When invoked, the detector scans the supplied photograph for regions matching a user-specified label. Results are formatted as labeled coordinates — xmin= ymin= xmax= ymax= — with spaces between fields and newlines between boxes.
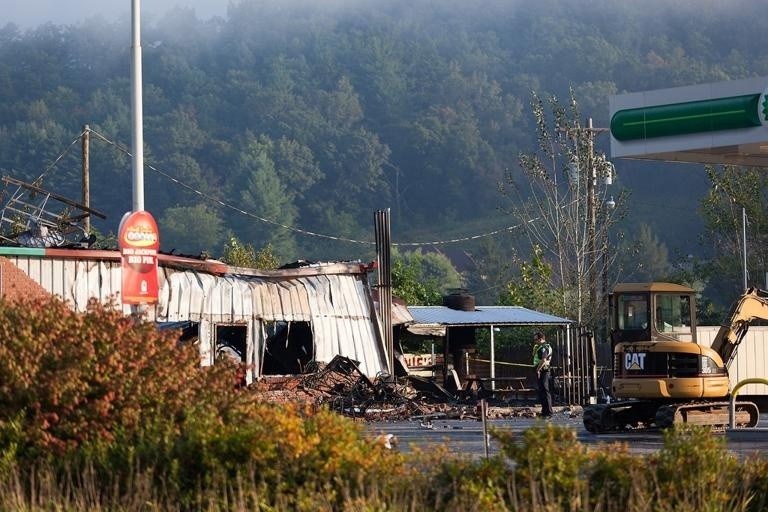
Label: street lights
xmin=600 ymin=194 xmax=616 ymax=302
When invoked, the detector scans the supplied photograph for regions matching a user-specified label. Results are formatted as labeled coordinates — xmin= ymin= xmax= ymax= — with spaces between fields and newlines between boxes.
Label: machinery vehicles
xmin=582 ymin=277 xmax=767 ymax=434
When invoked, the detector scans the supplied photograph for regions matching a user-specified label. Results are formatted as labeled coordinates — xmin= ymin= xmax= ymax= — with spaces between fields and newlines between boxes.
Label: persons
xmin=532 ymin=332 xmax=553 ymax=417
xmin=644 ymin=307 xmax=673 ymax=327
